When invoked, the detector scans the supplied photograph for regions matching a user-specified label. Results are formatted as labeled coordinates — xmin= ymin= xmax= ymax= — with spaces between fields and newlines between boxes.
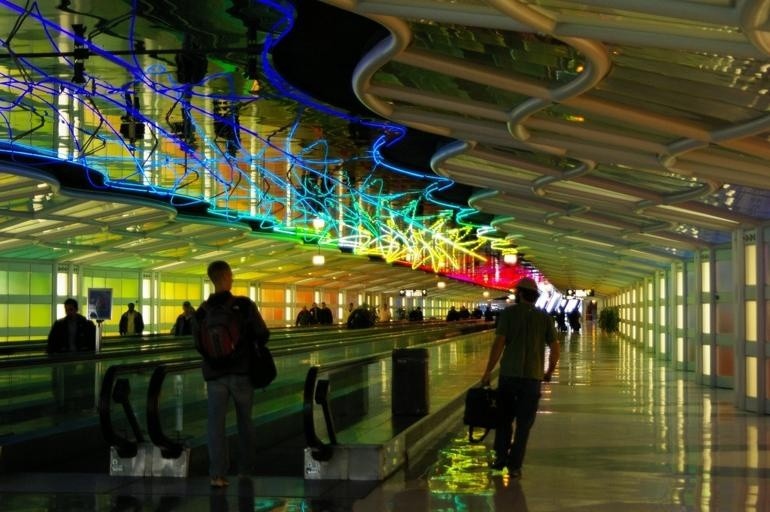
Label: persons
xmin=481 ymin=278 xmax=561 ymax=478
xmin=170 ymin=302 xmax=198 ymax=337
xmin=293 ymin=300 xmax=579 ymax=333
xmin=194 ymin=260 xmax=278 ymax=488
xmin=48 ymin=298 xmax=96 ymax=417
xmin=117 ymin=302 xmax=146 ymax=336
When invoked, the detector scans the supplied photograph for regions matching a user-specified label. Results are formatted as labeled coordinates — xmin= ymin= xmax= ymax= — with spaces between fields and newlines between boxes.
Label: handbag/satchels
xmin=246 ymin=347 xmax=276 ymax=389
xmin=463 ymin=384 xmax=501 ymax=429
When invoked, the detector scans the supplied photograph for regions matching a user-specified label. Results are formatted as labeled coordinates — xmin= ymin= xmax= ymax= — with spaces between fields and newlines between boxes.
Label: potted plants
xmin=599 ymin=306 xmax=620 ymax=339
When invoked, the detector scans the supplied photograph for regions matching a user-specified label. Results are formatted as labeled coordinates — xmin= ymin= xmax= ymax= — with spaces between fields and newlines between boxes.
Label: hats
xmin=518 ymin=277 xmax=538 ymax=291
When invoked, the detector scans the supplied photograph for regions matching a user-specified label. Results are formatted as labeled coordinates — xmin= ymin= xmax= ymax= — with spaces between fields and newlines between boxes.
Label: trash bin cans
xmin=393 ymin=349 xmax=428 ymax=417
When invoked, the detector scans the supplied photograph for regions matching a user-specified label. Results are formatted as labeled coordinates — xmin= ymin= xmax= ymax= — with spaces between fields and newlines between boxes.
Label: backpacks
xmin=201 ymin=298 xmax=245 ymax=363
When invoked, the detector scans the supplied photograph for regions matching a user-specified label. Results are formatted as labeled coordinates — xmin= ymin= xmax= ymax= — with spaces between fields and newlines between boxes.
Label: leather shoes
xmin=508 ymin=468 xmax=521 ymax=477
xmin=491 ymin=460 xmax=508 ymax=471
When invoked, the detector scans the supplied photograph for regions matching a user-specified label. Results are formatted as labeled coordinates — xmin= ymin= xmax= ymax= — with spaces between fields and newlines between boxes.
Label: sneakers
xmin=210 ymin=474 xmax=230 ymax=488
xmin=239 ymin=468 xmax=261 ymax=478
